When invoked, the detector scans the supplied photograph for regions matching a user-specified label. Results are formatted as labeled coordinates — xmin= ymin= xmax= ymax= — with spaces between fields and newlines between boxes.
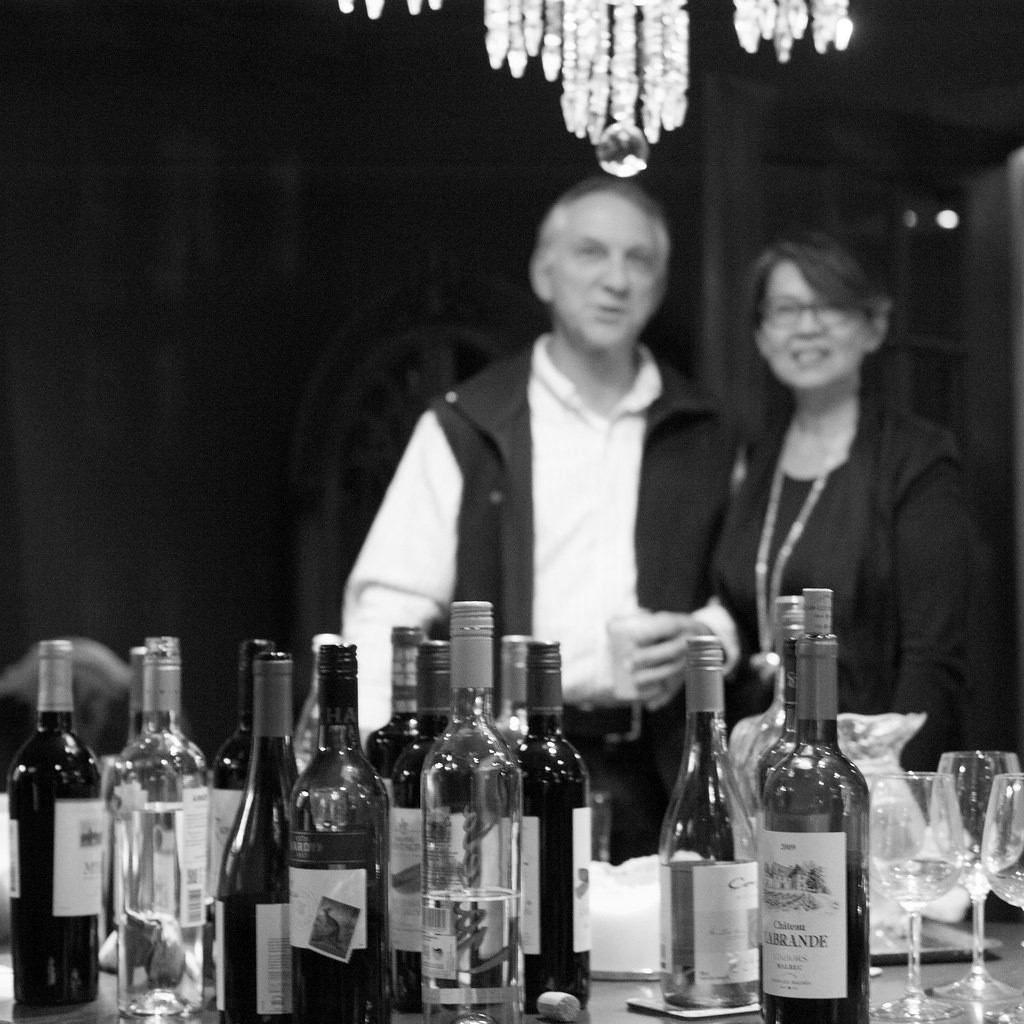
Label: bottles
xmin=658 ymin=589 xmax=870 ymax=1024
xmin=9 ymin=601 xmax=591 ymax=1024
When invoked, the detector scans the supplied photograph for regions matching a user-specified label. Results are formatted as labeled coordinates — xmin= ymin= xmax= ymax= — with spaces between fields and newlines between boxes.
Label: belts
xmin=559 ymin=696 xmax=686 ymax=747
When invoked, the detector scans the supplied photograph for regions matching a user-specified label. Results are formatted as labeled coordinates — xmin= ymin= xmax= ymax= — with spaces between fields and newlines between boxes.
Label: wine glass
xmin=924 ymin=752 xmax=1023 ymax=1007
xmin=981 ymin=773 xmax=1024 ymax=1024
xmin=867 ymin=772 xmax=964 ymax=1022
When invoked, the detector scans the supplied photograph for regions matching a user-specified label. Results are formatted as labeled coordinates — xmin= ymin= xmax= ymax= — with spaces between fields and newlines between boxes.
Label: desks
xmin=0 ymin=922 xmax=1024 ymax=1024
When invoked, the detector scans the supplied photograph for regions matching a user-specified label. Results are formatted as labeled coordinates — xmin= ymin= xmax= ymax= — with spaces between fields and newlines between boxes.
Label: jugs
xmin=838 ymin=711 xmax=926 ymax=943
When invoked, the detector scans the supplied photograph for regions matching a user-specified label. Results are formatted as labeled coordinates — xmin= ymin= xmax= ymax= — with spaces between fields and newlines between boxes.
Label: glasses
xmin=758 ymin=293 xmax=857 ymax=324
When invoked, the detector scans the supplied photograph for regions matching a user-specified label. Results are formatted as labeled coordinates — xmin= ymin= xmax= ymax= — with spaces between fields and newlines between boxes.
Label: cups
xmin=609 ymin=623 xmax=657 ymax=702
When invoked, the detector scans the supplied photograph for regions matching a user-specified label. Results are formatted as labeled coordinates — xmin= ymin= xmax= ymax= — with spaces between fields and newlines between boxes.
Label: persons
xmin=715 ymin=230 xmax=997 ymax=771
xmin=345 ymin=173 xmax=744 ymax=871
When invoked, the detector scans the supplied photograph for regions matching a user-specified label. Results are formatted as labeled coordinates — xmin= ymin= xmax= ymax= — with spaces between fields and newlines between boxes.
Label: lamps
xmin=338 ymin=0 xmax=854 ymax=178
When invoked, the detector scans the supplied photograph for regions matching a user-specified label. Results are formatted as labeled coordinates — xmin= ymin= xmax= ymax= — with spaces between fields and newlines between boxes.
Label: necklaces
xmin=754 ymin=434 xmax=847 ymax=683
